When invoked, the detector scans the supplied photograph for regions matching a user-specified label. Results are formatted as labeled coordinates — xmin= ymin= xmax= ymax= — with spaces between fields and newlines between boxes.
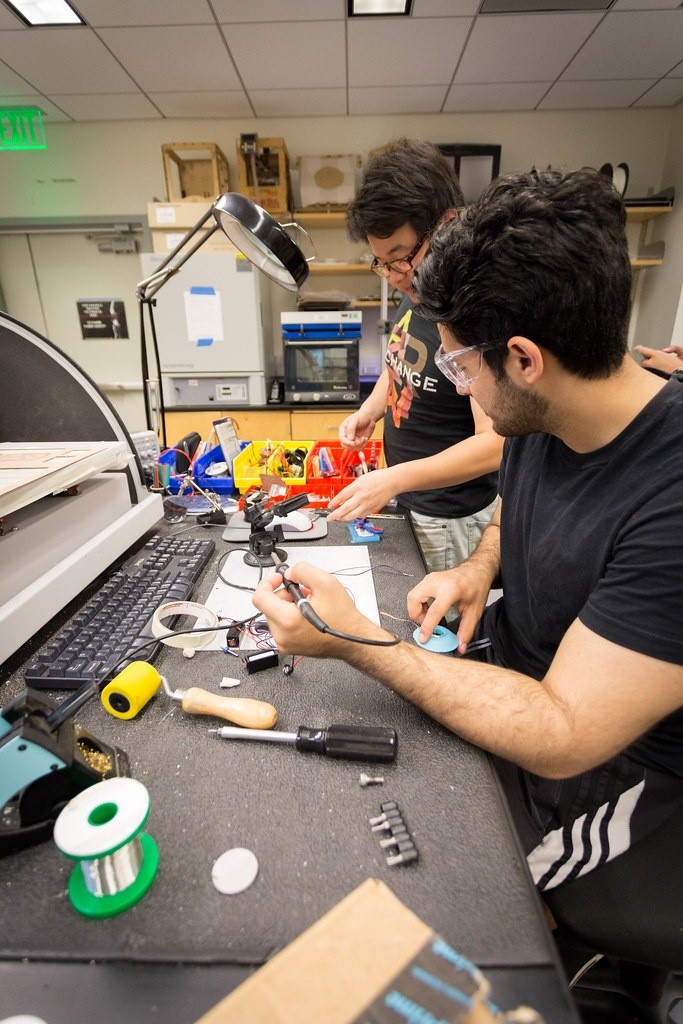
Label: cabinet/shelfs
xmin=270 ymin=213 xmax=400 ymax=307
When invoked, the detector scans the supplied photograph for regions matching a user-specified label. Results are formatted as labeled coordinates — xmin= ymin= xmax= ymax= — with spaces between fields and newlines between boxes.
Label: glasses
xmin=434 ymin=341 xmax=504 ymax=389
xmin=370 ymin=217 xmax=437 ymax=277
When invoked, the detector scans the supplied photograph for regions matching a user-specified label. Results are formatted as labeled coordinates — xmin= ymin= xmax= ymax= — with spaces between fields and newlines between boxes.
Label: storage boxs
xmin=297 ymin=153 xmax=356 ymax=206
xmin=161 ymin=142 xmax=230 ymax=204
xmin=157 ymin=437 xmax=384 ymax=516
xmin=235 ymin=136 xmax=293 ymax=213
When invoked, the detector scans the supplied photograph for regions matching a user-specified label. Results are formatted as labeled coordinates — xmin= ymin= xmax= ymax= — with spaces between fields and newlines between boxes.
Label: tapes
xmin=150 ymin=600 xmax=221 ymax=649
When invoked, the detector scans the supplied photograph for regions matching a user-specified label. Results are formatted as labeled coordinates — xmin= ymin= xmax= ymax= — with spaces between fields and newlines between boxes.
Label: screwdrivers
xmin=206 ymin=724 xmax=398 ymax=763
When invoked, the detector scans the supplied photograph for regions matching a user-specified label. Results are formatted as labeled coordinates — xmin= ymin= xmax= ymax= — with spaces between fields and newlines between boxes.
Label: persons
xmin=326 ymin=139 xmax=500 ymax=623
xmin=252 ymin=171 xmax=683 ymax=1024
xmin=632 ymin=343 xmax=683 ymax=382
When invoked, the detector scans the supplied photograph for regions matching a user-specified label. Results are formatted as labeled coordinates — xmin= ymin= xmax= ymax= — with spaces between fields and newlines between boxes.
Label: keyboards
xmin=25 ymin=535 xmax=217 ymax=689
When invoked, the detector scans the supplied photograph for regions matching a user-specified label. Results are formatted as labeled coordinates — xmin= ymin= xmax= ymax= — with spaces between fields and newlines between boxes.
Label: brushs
xmin=99 ymin=660 xmax=280 ymax=730
